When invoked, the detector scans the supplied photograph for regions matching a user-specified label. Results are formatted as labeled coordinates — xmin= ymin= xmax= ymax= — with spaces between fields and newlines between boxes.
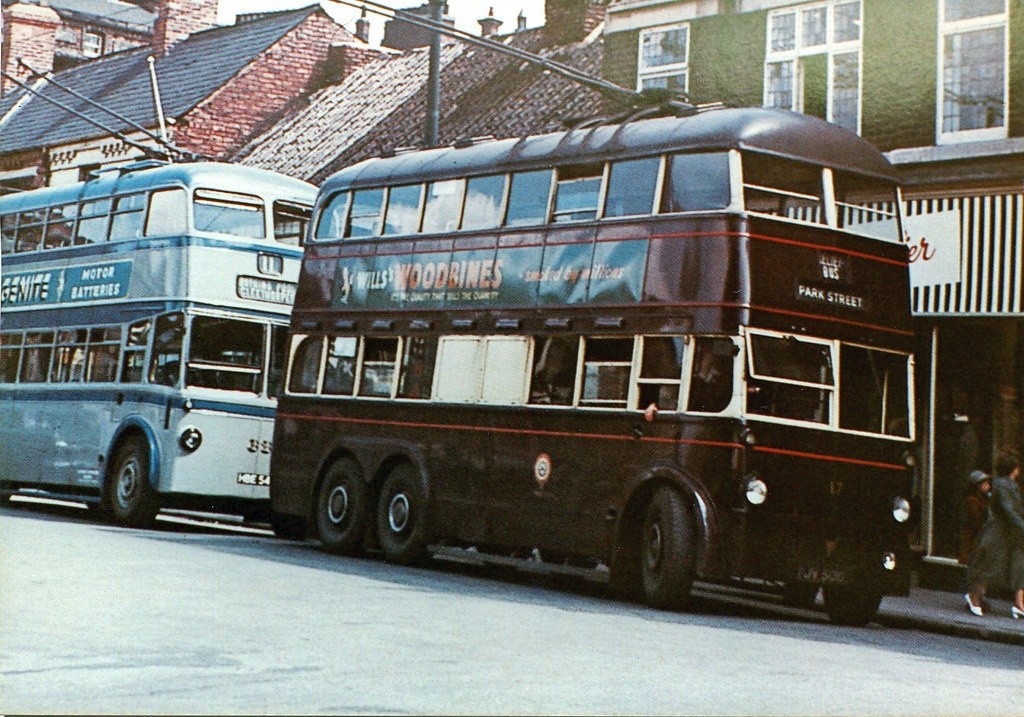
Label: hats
xmin=969 ymin=470 xmax=990 ymax=488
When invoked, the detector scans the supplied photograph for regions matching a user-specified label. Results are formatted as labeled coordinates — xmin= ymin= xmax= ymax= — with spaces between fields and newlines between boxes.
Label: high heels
xmin=965 ymin=593 xmax=983 ymax=616
xmin=1010 ymin=605 xmax=1024 ymax=620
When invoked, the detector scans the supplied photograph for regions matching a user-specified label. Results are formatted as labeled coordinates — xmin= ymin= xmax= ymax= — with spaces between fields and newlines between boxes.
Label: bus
xmin=262 ymin=88 xmax=925 ymax=632
xmin=1 ymin=160 xmax=324 ymax=529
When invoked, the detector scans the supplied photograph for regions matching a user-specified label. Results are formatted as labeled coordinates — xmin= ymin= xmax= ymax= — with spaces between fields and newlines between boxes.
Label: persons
xmin=966 ymin=447 xmax=1024 ymax=621
xmin=960 ymin=470 xmax=991 ymax=609
xmin=533 ymin=332 xmax=723 ymax=421
